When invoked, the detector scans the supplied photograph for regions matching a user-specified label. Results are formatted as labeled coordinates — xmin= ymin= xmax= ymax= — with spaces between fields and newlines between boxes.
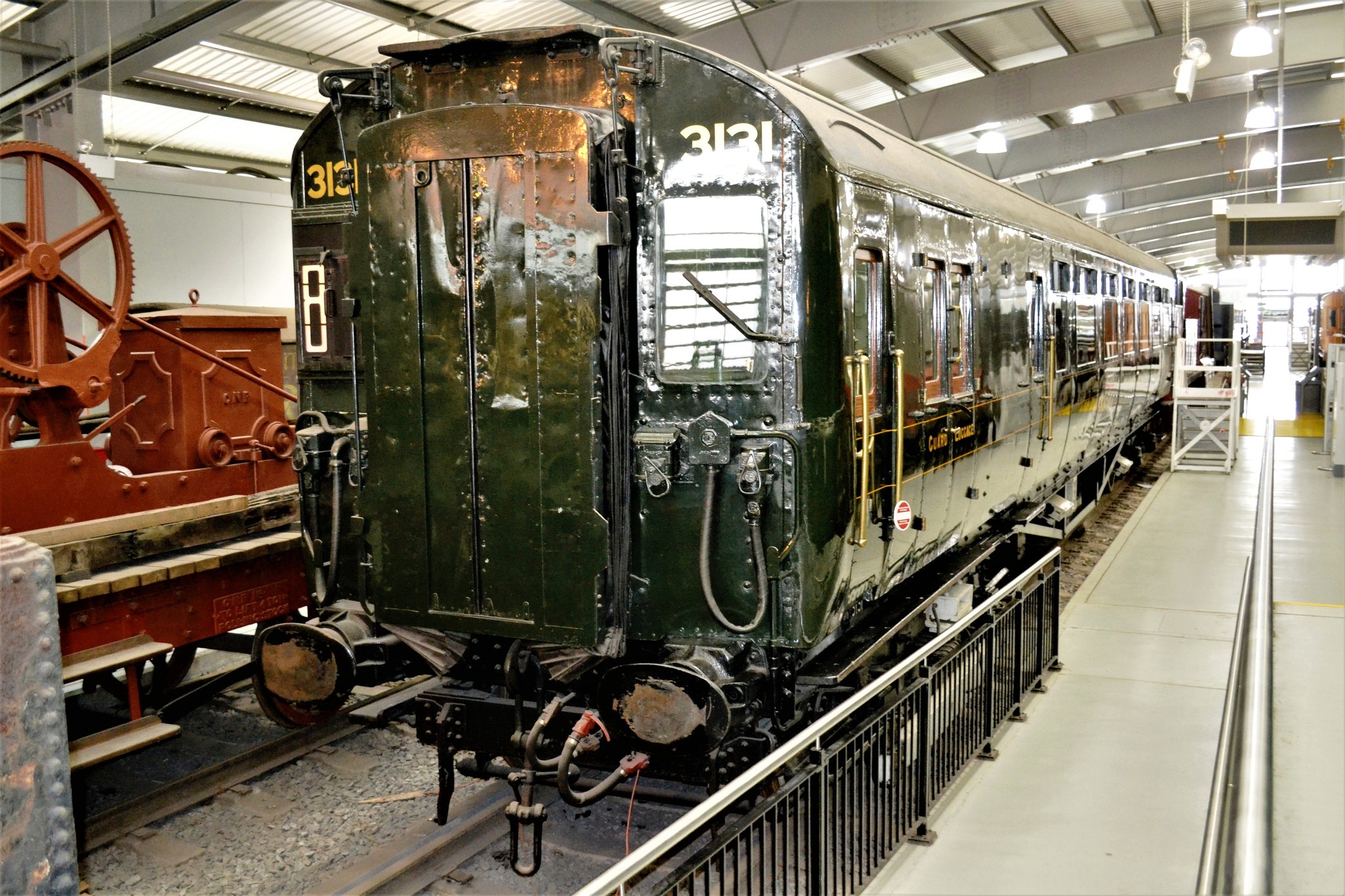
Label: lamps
xmin=1244 ymin=87 xmax=1276 ymax=129
xmin=1173 ymin=38 xmax=1211 ymax=102
xmin=1086 ymin=194 xmax=1106 ymax=214
xmin=1249 ymin=133 xmax=1274 ymax=170
xmin=1230 ymin=19 xmax=1273 ymax=56
xmin=976 ymin=131 xmax=1007 ymax=153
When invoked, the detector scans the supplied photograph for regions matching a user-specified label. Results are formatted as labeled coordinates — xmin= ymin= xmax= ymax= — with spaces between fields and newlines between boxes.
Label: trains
xmin=288 ymin=28 xmax=1246 ymax=878
